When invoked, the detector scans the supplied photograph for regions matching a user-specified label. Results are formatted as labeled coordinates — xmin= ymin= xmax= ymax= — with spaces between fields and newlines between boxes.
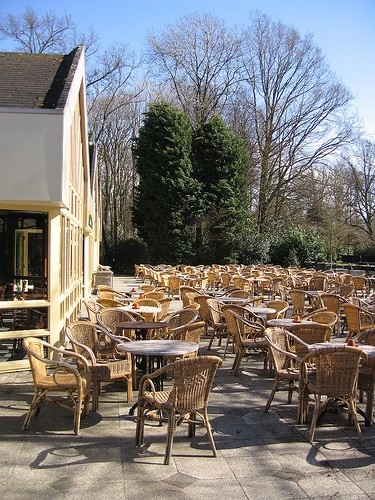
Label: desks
xmin=308 ymin=342 xmax=375 ymax=361
xmin=247 ymin=308 xmax=277 ymax=322
xmin=118 ymin=289 xmax=145 ymax=299
xmin=219 ymin=298 xmax=247 ymax=303
xmin=200 ymin=290 xmax=226 ymax=296
xmin=116 ymin=340 xmax=198 ymax=426
xmin=116 ymin=306 xmax=162 ymax=322
xmin=114 ymin=320 xmax=166 ymax=372
xmin=268 ymin=319 xmax=320 ymax=326
xmin=118 ymin=298 xmax=139 ymax=305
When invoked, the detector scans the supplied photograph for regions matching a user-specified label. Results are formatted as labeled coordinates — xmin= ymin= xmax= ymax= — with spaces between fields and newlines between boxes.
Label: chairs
xmin=0 ymin=263 xmax=375 ymax=465
